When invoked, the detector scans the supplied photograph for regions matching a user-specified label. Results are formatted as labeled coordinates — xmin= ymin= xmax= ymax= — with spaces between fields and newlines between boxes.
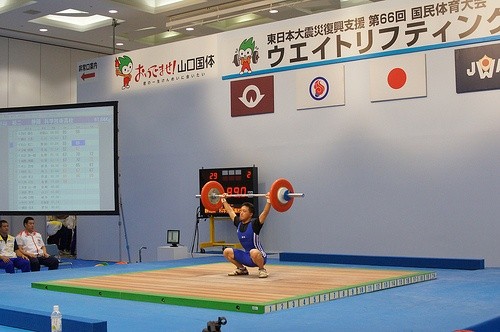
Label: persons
xmin=0 ymin=220 xmax=30 ymax=273
xmin=221 ymin=192 xmax=271 ymax=278
xmin=46 ymin=216 xmax=77 ymax=256
xmin=15 ymin=217 xmax=59 ymax=273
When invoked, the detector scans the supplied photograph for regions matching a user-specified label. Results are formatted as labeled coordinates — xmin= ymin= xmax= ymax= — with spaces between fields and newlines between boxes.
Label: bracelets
xmin=266 ymin=199 xmax=271 ymax=203
xmin=221 ymin=198 xmax=226 ymax=203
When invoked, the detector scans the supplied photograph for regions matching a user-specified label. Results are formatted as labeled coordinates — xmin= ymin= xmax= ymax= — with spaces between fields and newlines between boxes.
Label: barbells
xmin=194 ymin=178 xmax=306 ymax=214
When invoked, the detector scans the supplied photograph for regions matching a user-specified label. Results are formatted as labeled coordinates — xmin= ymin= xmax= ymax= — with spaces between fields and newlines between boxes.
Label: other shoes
xmin=70 ymin=253 xmax=74 ymax=256
xmin=59 ymin=249 xmax=69 ymax=255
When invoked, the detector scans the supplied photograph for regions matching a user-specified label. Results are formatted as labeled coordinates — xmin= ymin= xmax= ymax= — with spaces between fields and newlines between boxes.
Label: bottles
xmin=51 ymin=304 xmax=62 ymax=332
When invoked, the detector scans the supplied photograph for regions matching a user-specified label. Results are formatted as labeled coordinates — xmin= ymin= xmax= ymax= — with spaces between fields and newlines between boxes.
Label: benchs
xmin=14 ymin=244 xmax=72 ymax=271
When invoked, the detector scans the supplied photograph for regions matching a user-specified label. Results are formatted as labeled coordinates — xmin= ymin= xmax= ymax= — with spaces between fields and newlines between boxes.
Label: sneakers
xmin=258 ymin=268 xmax=269 ymax=278
xmin=228 ymin=267 xmax=249 ymax=276
